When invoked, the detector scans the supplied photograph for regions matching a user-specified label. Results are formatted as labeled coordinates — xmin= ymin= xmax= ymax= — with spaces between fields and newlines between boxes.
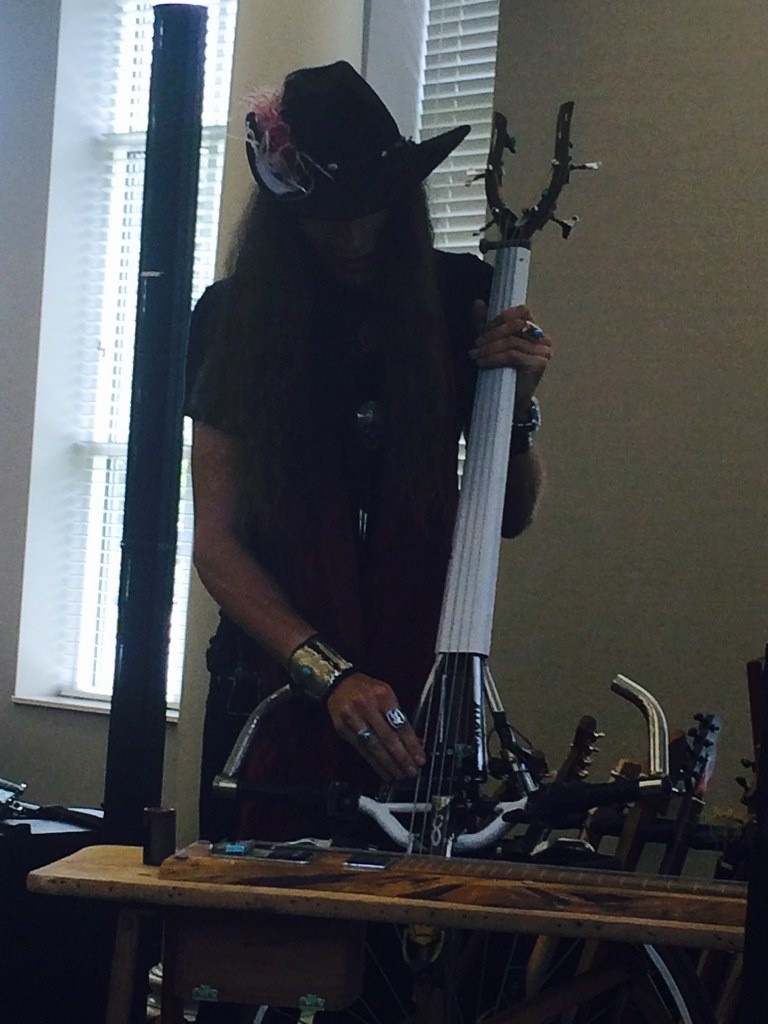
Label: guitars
xmin=355 ymin=97 xmax=601 ymax=985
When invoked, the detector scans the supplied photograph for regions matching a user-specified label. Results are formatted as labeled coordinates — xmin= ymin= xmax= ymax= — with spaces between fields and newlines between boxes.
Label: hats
xmin=243 ymin=61 xmax=470 ymax=220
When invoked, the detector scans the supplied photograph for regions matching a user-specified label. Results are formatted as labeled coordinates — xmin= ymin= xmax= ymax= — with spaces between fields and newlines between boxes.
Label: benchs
xmin=24 ymin=842 xmax=746 ymax=1024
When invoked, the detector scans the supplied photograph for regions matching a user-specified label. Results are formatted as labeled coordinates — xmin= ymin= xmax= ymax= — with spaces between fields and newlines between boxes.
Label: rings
xmin=519 ymin=321 xmax=544 ymax=343
xmin=357 ymin=726 xmax=373 ymax=742
xmin=387 ymin=707 xmax=409 ymax=731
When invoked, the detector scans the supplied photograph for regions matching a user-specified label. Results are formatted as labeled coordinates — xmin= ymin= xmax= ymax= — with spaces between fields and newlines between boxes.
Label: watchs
xmin=512 ymin=393 xmax=543 ymax=433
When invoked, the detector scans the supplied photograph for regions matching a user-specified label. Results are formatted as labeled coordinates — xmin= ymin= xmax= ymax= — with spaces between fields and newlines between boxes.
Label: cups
xmin=142 ymin=806 xmax=177 ymax=865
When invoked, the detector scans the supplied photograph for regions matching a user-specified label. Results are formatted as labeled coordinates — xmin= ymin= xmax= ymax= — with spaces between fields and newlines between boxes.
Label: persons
xmin=177 ymin=62 xmax=549 ymax=840
